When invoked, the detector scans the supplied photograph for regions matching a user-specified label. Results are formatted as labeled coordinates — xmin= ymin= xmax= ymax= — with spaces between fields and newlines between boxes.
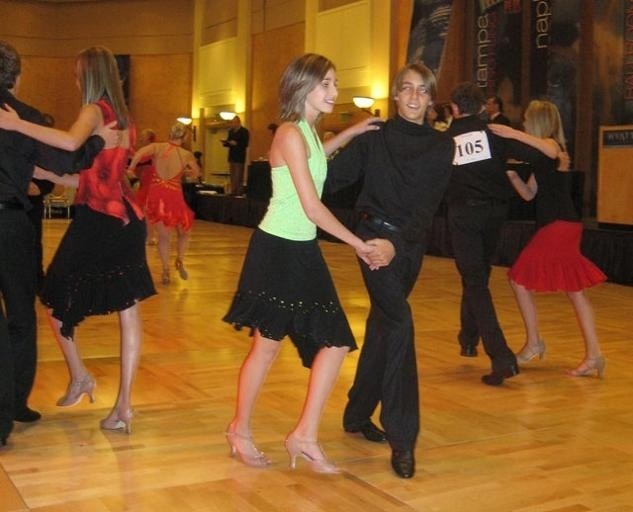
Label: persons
xmin=0 ymin=44 xmax=159 ymax=433
xmin=438 ymin=81 xmax=571 ymax=387
xmin=484 ymin=99 xmax=610 ymax=381
xmin=219 ymin=51 xmax=386 ymax=476
xmin=21 ymin=114 xmax=251 ymax=303
xmin=423 ymin=103 xmax=454 ymax=133
xmin=322 ymin=62 xmax=457 ymax=479
xmin=0 ymin=42 xmax=125 ymax=448
xmin=484 ymin=94 xmax=512 ymax=128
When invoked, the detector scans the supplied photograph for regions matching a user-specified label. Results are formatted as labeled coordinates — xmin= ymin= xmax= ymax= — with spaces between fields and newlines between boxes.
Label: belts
xmin=363 ymin=210 xmax=406 ymax=235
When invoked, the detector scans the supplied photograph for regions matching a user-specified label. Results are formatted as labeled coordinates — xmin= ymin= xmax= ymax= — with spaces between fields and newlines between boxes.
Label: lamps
xmin=352 ymin=96 xmax=379 ymax=117
xmin=177 ymin=118 xmax=195 ymax=142
xmin=219 ymin=112 xmax=239 ymax=121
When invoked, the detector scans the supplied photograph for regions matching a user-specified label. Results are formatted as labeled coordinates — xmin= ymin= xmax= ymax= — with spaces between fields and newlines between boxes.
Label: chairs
xmin=43 ymin=184 xmax=70 ymax=219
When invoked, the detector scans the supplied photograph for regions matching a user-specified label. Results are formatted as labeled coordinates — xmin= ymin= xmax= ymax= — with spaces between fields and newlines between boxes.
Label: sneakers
xmin=160 ymin=264 xmax=170 ymax=284
xmin=173 ymin=259 xmax=188 ymax=281
xmin=15 ymin=406 xmax=41 ymax=425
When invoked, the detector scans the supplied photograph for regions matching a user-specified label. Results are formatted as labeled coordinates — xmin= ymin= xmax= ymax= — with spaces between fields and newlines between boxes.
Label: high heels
xmin=52 ymin=372 xmax=100 ymax=409
xmin=566 ymin=354 xmax=607 ymax=381
xmin=220 ymin=420 xmax=274 ymax=469
xmin=282 ymin=431 xmax=344 ymax=476
xmin=97 ymin=403 xmax=134 ymax=436
xmin=515 ymin=339 xmax=546 ymax=365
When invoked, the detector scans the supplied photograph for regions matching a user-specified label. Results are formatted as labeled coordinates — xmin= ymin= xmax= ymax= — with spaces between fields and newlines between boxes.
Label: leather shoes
xmin=459 ymin=344 xmax=476 ymax=356
xmin=389 ymin=446 xmax=417 ymax=480
xmin=341 ymin=416 xmax=388 ymax=443
xmin=480 ymin=364 xmax=518 ymax=388
